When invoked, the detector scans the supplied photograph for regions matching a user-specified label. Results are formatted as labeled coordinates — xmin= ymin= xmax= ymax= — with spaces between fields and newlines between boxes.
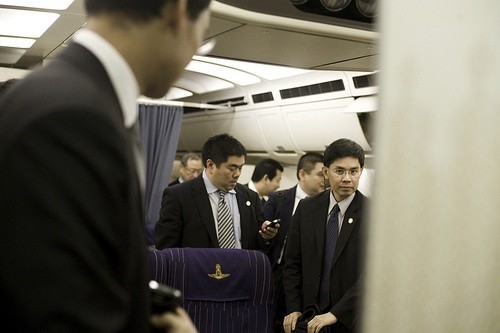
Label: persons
xmin=154 ymin=134 xmax=280 ymax=251
xmin=168 ymin=152 xmax=203 ymax=187
xmin=241 ymin=139 xmax=370 ymax=333
xmin=0 ymin=0 xmax=214 ymax=333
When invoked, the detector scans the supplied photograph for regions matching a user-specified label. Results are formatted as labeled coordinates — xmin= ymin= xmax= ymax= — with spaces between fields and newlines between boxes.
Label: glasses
xmin=185 ymin=167 xmax=203 ymax=174
xmin=332 ymin=169 xmax=362 ymax=177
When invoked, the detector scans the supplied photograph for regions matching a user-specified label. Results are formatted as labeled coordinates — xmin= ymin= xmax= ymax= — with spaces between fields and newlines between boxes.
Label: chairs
xmin=148 ymin=246 xmax=167 ymax=287
xmin=162 ymin=247 xmax=274 ymax=333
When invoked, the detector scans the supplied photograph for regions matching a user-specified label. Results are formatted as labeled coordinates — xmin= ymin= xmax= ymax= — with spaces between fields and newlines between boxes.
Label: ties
xmin=217 ymin=190 xmax=236 ymax=250
xmin=319 ymin=204 xmax=341 ymax=312
xmin=129 ymin=118 xmax=147 ymax=228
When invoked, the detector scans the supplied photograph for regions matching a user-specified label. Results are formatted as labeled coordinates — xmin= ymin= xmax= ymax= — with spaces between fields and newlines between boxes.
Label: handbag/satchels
xmin=274 ymin=304 xmax=330 ymax=333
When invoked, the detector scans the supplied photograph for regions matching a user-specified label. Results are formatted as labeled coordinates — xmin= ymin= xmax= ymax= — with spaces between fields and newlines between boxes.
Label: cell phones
xmin=262 ymin=219 xmax=281 ymax=233
xmin=148 ymin=280 xmax=181 ymax=333
